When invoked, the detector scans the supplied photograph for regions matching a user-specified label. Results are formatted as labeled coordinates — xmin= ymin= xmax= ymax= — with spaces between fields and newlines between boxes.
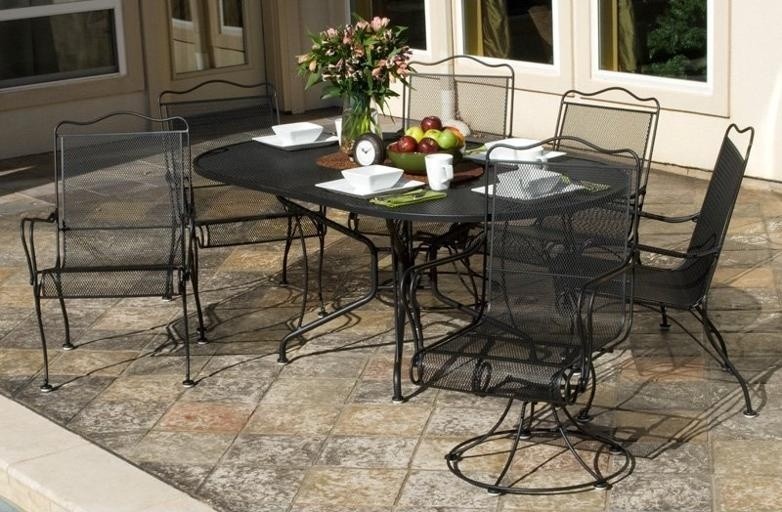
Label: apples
xmin=389 ymin=116 xmax=465 ymax=154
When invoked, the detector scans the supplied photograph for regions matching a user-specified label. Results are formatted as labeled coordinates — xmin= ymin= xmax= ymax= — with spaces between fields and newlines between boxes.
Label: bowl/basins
xmin=339 ymin=164 xmax=403 ymax=191
xmin=499 ymin=167 xmax=562 ymax=196
xmin=386 ymin=141 xmax=466 ymax=176
xmin=483 ymin=136 xmax=542 ymax=160
xmin=271 ymin=121 xmax=323 ymax=144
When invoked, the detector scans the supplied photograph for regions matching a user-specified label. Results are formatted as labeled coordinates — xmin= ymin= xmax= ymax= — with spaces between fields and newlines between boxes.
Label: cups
xmin=334 ymin=117 xmax=345 ymax=146
xmin=424 ymin=153 xmax=454 ymax=191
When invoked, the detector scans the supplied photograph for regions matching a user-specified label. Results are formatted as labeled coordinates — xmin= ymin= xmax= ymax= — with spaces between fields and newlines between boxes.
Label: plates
xmin=251 ymin=133 xmax=340 ymax=153
xmin=314 ymin=176 xmax=424 ymax=201
xmin=464 ymin=146 xmax=567 ymax=166
xmin=471 ymin=184 xmax=586 ymax=203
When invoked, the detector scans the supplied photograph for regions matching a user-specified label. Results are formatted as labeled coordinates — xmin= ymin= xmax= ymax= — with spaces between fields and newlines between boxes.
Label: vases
xmin=338 ymin=89 xmax=387 ymax=155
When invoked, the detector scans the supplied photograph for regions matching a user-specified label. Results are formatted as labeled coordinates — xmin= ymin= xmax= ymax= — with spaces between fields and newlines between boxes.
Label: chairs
xmin=347 ymin=49 xmax=520 ymax=313
xmin=17 ymin=104 xmax=212 ymax=393
xmin=157 ymin=73 xmax=332 ymax=346
xmin=405 ymin=133 xmax=644 ymax=498
xmin=555 ymin=119 xmax=765 ymax=422
xmin=447 ymin=77 xmax=664 ymax=339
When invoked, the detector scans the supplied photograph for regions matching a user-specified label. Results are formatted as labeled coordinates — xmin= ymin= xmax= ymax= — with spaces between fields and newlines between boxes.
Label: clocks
xmin=353 ymin=132 xmax=387 ymax=168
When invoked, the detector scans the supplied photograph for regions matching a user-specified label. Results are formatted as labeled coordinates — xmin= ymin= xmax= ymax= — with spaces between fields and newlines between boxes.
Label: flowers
xmin=292 ymin=12 xmax=415 ymax=145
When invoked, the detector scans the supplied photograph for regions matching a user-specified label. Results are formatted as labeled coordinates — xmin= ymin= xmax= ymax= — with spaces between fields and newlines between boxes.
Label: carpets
xmin=2 ymin=102 xmax=782 ymax=512
